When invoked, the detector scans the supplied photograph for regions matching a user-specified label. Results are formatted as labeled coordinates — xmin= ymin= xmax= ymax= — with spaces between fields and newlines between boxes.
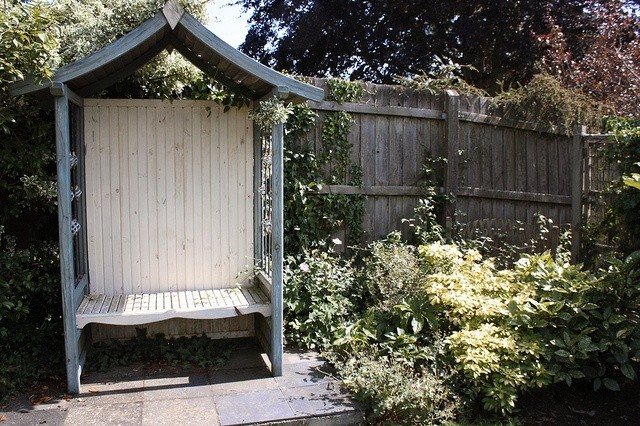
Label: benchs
xmin=73 ymin=268 xmax=277 ymax=328
